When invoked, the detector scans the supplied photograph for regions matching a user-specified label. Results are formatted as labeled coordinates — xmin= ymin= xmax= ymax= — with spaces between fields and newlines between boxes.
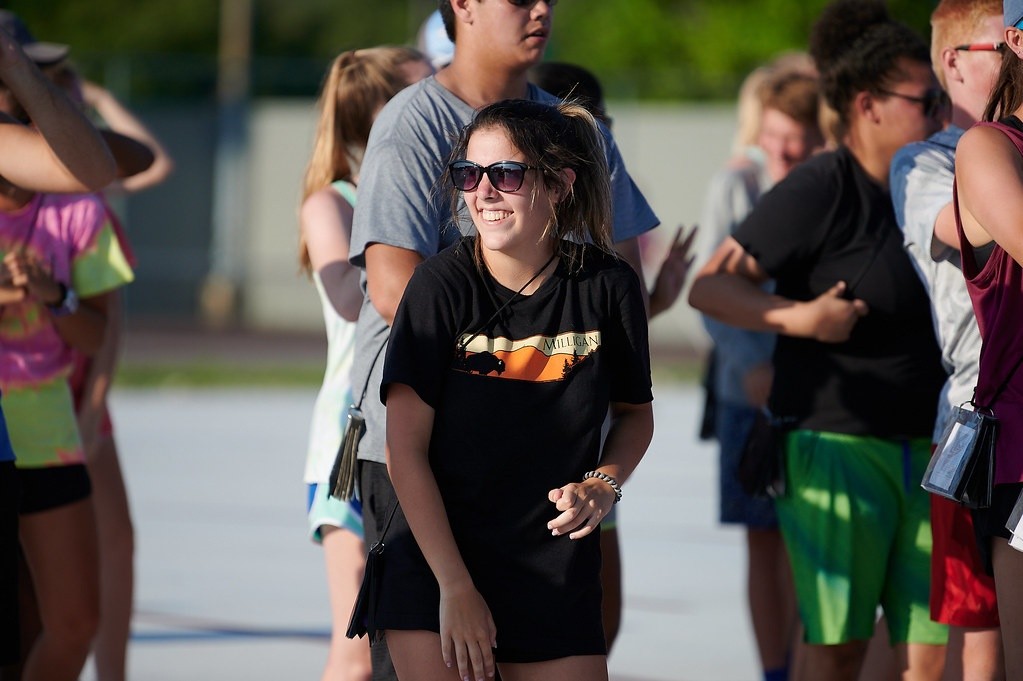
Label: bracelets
xmin=583 ymin=471 xmax=622 ymax=504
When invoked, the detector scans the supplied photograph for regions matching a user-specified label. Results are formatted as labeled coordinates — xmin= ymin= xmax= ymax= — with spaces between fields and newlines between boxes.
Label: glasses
xmin=449 ymin=160 xmax=548 ymax=193
xmin=873 ymin=88 xmax=944 ymax=118
xmin=954 ymin=42 xmax=1009 ymax=62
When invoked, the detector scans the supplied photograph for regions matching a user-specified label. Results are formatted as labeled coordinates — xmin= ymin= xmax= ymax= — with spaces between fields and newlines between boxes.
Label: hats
xmin=1004 ymin=0 xmax=1023 ymax=29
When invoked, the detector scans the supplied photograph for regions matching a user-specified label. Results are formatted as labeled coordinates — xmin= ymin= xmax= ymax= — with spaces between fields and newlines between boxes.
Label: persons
xmin=295 ymin=47 xmax=436 ymax=681
xmin=372 ymin=100 xmax=654 ymax=681
xmin=527 ymin=62 xmax=698 ymax=325
xmin=347 ymin=0 xmax=660 ymax=681
xmin=687 ymin=23 xmax=945 ymax=681
xmin=420 ymin=9 xmax=454 ymax=69
xmin=889 ymin=0 xmax=1004 ymax=681
xmin=952 ymin=0 xmax=1023 ymax=681
xmin=0 ymin=8 xmax=170 ymax=681
xmin=704 ymin=0 xmax=902 ymax=681
